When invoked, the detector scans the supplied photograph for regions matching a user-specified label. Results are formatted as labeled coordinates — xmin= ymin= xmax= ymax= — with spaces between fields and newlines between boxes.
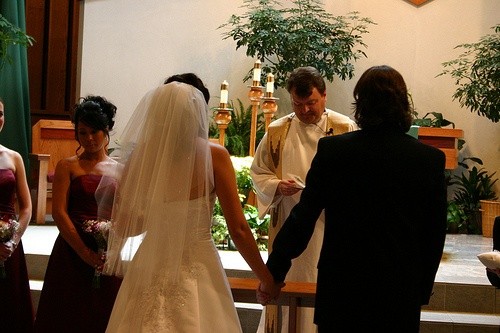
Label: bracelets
xmin=10 ymin=240 xmax=17 ymax=249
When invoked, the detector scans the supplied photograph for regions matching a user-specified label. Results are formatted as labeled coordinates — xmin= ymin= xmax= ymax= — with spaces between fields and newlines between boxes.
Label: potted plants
xmin=411 ymin=112 xmax=465 ymax=169
xmin=436 ymin=22 xmax=500 ymax=245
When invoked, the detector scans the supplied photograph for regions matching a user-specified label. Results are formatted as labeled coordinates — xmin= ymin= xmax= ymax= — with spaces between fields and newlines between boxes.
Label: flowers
xmin=0 ymin=215 xmax=21 ymax=268
xmin=84 ymin=218 xmax=112 ymax=281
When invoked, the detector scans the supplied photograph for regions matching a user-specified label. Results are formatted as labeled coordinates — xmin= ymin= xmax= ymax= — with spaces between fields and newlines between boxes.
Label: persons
xmin=35 ymin=67 xmax=448 ymax=333
xmin=486 ymin=216 xmax=500 ymax=290
xmin=0 ymin=100 xmax=34 ymax=333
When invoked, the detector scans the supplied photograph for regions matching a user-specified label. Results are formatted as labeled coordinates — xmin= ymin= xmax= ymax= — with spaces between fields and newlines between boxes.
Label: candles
xmin=266 ymin=73 xmax=274 ymax=98
xmin=220 ymin=80 xmax=229 ymax=108
xmin=253 ymin=58 xmax=262 ymax=87
